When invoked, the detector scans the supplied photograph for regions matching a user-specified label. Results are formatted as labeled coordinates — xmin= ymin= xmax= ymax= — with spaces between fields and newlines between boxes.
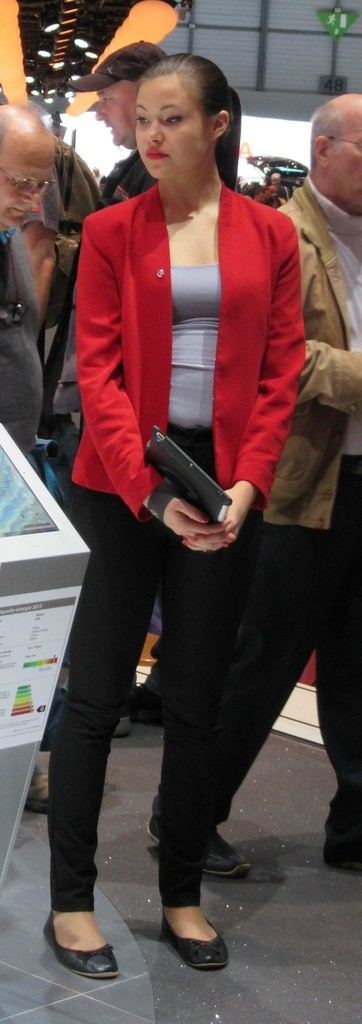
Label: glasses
xmin=0 ymin=167 xmax=57 ymax=196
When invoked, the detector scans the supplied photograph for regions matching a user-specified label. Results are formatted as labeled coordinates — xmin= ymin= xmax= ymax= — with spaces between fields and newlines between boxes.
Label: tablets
xmin=143 ymin=425 xmax=233 ymax=525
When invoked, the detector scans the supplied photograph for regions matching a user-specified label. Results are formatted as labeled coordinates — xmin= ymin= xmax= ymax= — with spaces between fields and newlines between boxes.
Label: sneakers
xmin=147 ymin=824 xmax=254 ymax=877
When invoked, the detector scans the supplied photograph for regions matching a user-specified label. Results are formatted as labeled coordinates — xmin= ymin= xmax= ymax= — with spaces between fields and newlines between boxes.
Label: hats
xmin=67 ymin=41 xmax=168 ymax=92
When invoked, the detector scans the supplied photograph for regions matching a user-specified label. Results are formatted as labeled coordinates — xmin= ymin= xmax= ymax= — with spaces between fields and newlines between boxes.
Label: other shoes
xmin=113 ymin=702 xmax=132 ymax=737
xmin=161 ymin=913 xmax=228 ymax=968
xmin=42 ymin=911 xmax=119 ymax=977
xmin=133 ymin=682 xmax=164 ymax=722
xmin=24 ymin=765 xmax=50 ymax=813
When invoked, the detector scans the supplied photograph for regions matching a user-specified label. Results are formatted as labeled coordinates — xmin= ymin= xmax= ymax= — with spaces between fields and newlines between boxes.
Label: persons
xmin=146 ymin=94 xmax=362 ymax=874
xmin=36 ymin=39 xmax=167 ymax=543
xmin=236 ymin=171 xmax=297 ymax=212
xmin=45 ymin=53 xmax=308 ymax=980
xmin=0 ymin=79 xmax=106 ymax=814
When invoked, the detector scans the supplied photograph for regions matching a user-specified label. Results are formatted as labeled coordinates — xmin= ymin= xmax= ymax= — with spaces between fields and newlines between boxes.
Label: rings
xmin=204 ymin=549 xmax=207 ymax=553
